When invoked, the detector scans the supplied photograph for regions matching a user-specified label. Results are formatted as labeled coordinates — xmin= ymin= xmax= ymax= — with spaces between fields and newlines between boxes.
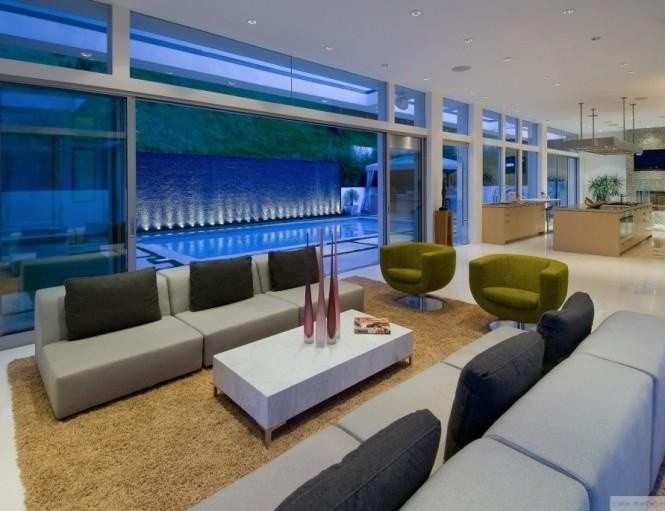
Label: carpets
xmin=8 ymin=274 xmax=498 ymax=511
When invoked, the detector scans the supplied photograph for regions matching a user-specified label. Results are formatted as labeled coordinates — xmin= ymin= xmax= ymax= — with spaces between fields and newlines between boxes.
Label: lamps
xmin=566 ymin=97 xmax=643 ymax=156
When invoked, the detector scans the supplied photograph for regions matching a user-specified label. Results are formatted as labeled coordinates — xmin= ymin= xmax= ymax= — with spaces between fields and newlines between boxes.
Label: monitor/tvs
xmin=633 ymin=149 xmax=665 ymax=171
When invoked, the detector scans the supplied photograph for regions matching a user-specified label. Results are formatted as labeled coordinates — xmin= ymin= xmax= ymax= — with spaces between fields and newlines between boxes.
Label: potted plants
xmin=585 ymin=172 xmax=625 ymax=204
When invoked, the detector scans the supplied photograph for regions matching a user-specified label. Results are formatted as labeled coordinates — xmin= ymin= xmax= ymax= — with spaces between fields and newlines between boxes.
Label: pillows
xmin=268 ymin=246 xmax=319 ymax=292
xmin=64 ymin=266 xmax=162 ymax=341
xmin=272 ymin=290 xmax=594 ymax=511
xmin=189 ymin=255 xmax=254 ymax=312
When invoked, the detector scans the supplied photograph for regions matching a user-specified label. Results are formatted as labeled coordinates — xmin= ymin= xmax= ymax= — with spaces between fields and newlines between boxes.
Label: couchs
xmin=34 ymin=247 xmax=364 ymax=421
xmin=185 ymin=310 xmax=665 ymax=511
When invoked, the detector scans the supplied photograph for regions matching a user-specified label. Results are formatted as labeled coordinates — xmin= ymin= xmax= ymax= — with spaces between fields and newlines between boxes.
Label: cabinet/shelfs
xmin=618 ymin=206 xmax=653 ymax=254
xmin=482 ymin=201 xmax=546 ymax=245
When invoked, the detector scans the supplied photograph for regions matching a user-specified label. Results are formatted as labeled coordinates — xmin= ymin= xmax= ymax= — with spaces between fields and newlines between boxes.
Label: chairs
xmin=379 ymin=241 xmax=456 ymax=311
xmin=469 ymin=253 xmax=568 ymax=332
